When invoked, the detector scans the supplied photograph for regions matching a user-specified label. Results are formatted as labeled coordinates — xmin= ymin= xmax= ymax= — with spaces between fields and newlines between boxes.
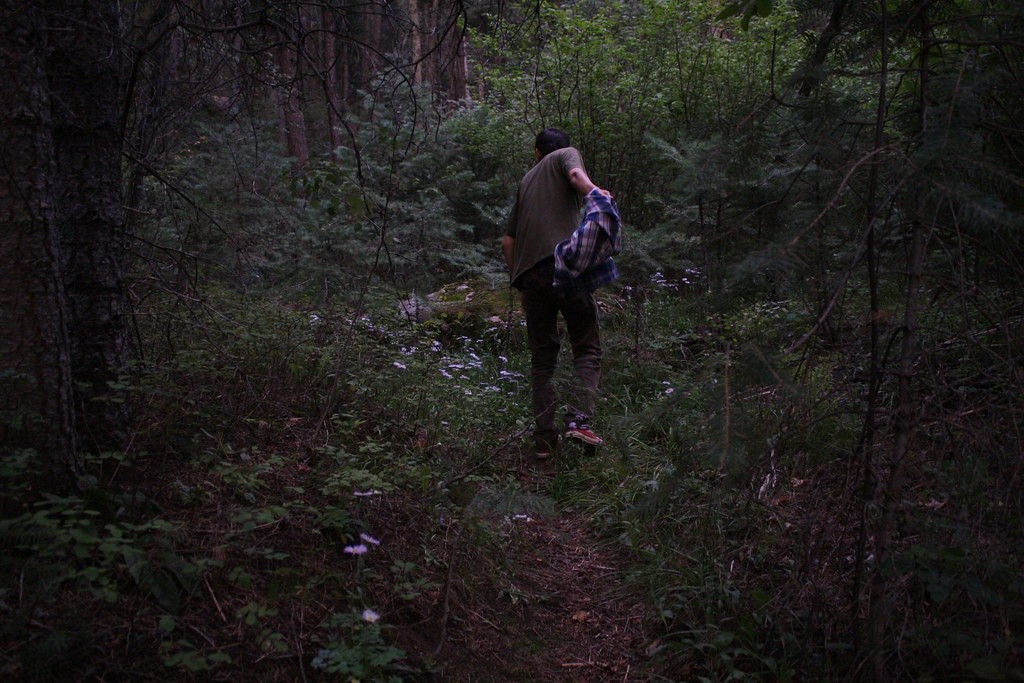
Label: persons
xmin=500 ymin=128 xmax=611 ymax=458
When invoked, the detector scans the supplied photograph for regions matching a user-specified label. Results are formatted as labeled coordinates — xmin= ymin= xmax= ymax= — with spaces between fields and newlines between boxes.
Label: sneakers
xmin=535 ymin=433 xmax=563 ymax=457
xmin=563 ymin=420 xmax=603 ymax=449
